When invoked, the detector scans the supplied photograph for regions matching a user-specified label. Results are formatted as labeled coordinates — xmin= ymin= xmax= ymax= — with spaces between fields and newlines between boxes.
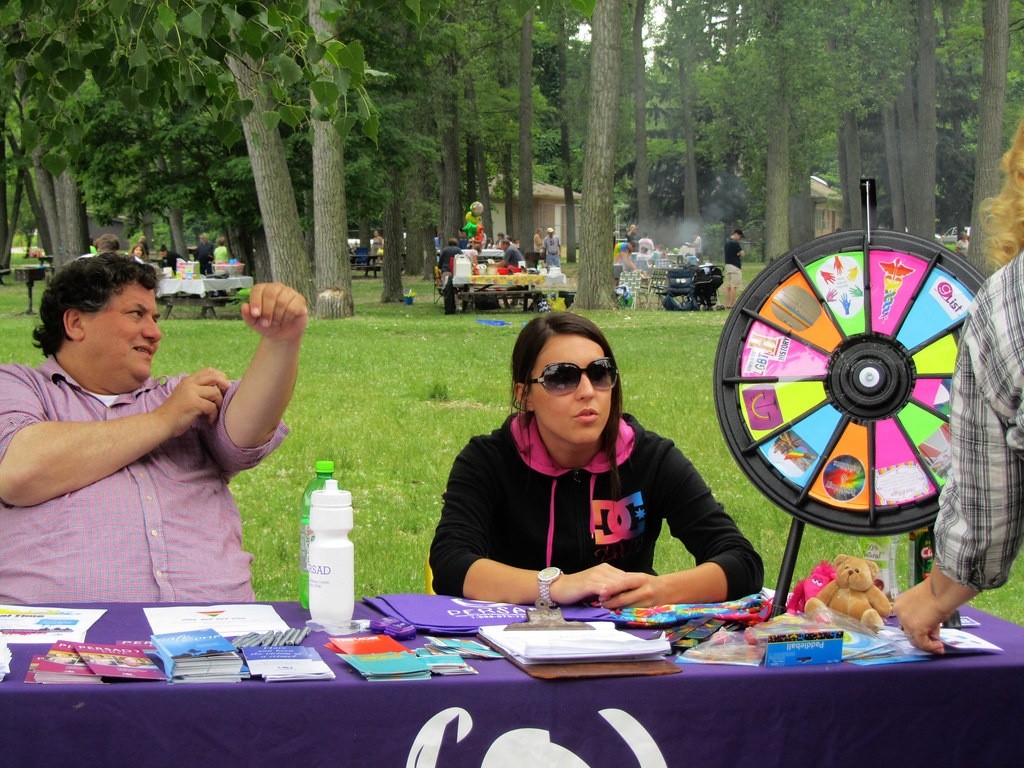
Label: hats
xmin=548 ymin=228 xmax=553 ymax=232
xmin=735 ymin=230 xmax=745 ymax=237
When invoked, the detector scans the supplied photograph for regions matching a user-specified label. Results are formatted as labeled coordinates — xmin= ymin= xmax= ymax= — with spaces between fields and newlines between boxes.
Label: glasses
xmin=528 ymin=357 xmax=619 ymax=396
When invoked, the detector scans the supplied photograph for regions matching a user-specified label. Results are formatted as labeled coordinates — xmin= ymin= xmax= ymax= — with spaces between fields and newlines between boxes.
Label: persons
xmin=612 ymin=223 xmax=702 ymax=264
xmin=955 ymin=231 xmax=969 ymax=255
xmin=436 ymin=227 xmax=562 ymax=315
xmin=88 ymin=234 xmax=230 ymax=298
xmin=0 ymin=247 xmax=308 ymax=604
xmin=426 ymin=312 xmax=763 ymax=610
xmin=351 ymin=230 xmax=383 ymax=278
xmin=892 ymin=120 xmax=1024 ymax=653
xmin=721 ymin=228 xmax=747 ymax=307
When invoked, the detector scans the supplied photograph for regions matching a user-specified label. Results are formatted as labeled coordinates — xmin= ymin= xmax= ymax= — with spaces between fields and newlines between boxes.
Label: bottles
xmin=860 ymin=534 xmax=900 ymax=617
xmin=307 ymin=478 xmax=354 ymax=624
xmin=908 ymin=527 xmax=934 ymax=592
xmin=299 ymin=460 xmax=344 ymax=610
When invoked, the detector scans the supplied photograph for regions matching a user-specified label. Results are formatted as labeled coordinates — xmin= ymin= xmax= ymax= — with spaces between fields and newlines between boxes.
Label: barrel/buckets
xmin=455 ymin=254 xmax=471 ymax=277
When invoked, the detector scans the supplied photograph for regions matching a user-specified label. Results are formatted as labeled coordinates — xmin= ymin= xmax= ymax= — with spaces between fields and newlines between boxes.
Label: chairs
xmin=662 ymin=268 xmax=694 ymax=309
xmin=433 ymin=264 xmax=451 ymax=304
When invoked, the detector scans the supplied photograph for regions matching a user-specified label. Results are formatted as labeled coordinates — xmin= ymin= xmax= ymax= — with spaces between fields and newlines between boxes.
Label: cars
xmin=941 ymin=225 xmax=970 ymax=243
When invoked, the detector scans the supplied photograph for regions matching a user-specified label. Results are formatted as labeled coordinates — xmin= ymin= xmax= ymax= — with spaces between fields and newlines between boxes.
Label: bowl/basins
xmin=498 ymin=268 xmax=509 ymax=275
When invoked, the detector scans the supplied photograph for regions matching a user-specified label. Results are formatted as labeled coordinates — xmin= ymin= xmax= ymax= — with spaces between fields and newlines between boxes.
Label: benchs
xmin=350 ymin=264 xmax=381 ymax=278
xmin=457 ymin=290 xmax=542 ymax=311
xmin=158 ymin=295 xmax=246 ymax=320
xmin=546 ymin=290 xmax=576 ymax=308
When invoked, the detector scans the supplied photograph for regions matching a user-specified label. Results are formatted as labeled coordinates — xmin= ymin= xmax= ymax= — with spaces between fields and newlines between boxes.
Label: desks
xmin=455 ymin=272 xmax=543 ymax=308
xmin=0 ymin=596 xmax=1024 ymax=768
xmin=349 ymin=255 xmax=383 ymax=278
xmin=157 ymin=275 xmax=253 ymax=320
xmin=437 ymin=249 xmax=504 ymax=264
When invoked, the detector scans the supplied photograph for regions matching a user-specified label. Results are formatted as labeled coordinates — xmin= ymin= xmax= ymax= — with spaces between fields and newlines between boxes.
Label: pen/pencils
xmin=240 ymin=634 xmax=265 ymax=648
xmin=294 ymin=626 xmax=312 ymax=646
xmin=261 ymin=631 xmax=281 ymax=647
xmin=286 ymin=629 xmax=301 ymax=646
xmin=277 ymin=628 xmax=296 ymax=647
xmin=235 ymin=633 xmax=259 ymax=647
xmin=249 ymin=630 xmax=274 ymax=647
xmin=232 ymin=632 xmax=255 ymax=646
xmin=272 ymin=632 xmax=283 ymax=647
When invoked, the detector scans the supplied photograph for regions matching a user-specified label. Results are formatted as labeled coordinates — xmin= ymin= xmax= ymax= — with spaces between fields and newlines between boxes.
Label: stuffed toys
xmin=787 ymin=559 xmax=838 ymax=616
xmin=804 ymin=554 xmax=891 ymax=634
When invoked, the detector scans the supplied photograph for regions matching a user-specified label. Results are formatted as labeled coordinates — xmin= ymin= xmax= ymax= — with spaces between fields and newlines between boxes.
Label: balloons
xmin=460 ymin=201 xmax=485 ymax=243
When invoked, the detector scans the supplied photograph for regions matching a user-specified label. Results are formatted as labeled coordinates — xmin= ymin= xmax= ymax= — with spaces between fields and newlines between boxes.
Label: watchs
xmin=537 ymin=567 xmax=564 ymax=606
